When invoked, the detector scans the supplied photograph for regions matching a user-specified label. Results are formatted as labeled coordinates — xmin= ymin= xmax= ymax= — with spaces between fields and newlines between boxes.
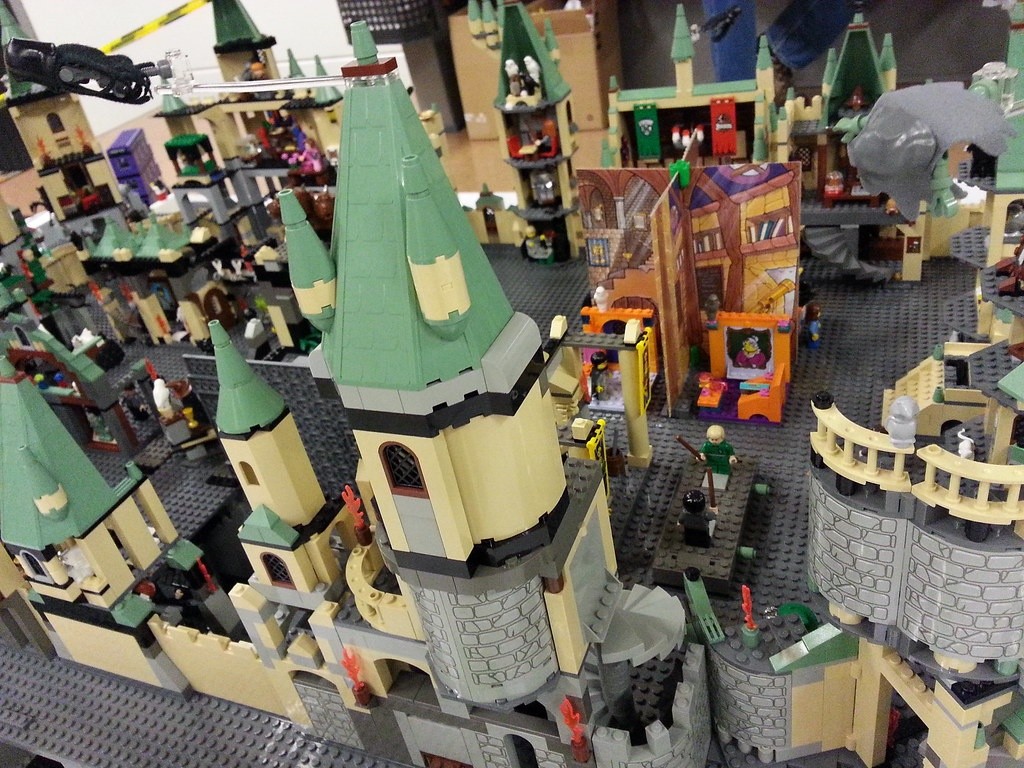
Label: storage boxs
xmin=448 ymin=0 xmax=624 ymax=141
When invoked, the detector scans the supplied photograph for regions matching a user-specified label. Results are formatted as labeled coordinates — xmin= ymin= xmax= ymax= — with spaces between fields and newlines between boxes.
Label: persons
xmin=736 ymin=338 xmax=765 ymax=369
xmin=298 ymin=139 xmax=324 ymax=177
xmin=159 ymin=566 xmax=210 ymax=633
xmin=704 ymin=0 xmax=850 ymax=115
xmin=242 ymin=63 xmax=272 ymax=100
xmin=590 ymin=352 xmax=614 ymax=401
xmin=696 ymin=425 xmax=738 ymax=475
xmin=679 ymin=489 xmax=718 ymax=549
xmin=535 ymin=120 xmax=557 ymax=153
xmin=804 ymin=303 xmax=821 ymax=351
xmin=119 ymin=382 xmax=150 ymax=421
